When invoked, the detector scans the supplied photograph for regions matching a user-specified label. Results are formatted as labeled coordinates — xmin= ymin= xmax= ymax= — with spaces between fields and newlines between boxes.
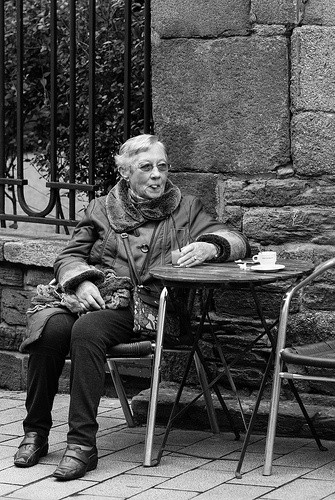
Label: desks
xmin=150 ymin=256 xmax=327 ymax=478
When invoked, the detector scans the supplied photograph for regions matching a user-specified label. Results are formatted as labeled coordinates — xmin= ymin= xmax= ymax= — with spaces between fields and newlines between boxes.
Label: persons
xmin=14 ymin=133 xmax=250 ymax=478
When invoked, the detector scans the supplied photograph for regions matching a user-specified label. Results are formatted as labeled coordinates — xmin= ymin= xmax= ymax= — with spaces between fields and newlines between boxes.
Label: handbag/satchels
xmin=132 ymin=287 xmax=182 ymax=345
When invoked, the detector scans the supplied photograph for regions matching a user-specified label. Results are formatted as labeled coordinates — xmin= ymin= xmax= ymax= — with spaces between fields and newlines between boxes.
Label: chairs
xmin=49 ymin=278 xmax=220 ymax=467
xmin=263 ymin=257 xmax=335 ymax=476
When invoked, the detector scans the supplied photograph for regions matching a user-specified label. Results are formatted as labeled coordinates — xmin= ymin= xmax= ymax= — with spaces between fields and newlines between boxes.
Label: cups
xmin=170 ymin=227 xmax=190 ymax=268
xmin=252 ymin=251 xmax=277 ymax=268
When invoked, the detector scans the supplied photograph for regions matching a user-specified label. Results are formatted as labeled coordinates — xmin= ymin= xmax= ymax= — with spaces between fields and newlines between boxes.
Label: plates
xmin=251 ymin=263 xmax=285 ymax=272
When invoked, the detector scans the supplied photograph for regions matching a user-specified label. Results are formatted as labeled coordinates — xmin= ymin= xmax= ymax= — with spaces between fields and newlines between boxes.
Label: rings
xmin=194 ymin=256 xmax=197 ymax=260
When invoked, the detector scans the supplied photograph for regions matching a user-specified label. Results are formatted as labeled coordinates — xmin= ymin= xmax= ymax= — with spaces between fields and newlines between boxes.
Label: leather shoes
xmin=52 ymin=444 xmax=98 ymax=480
xmin=13 ymin=431 xmax=48 ymax=468
